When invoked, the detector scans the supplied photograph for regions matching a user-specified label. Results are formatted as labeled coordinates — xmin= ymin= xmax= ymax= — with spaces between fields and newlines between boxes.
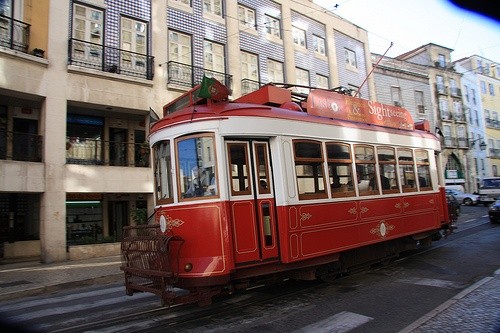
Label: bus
xmin=120 ymin=75 xmax=458 ymax=304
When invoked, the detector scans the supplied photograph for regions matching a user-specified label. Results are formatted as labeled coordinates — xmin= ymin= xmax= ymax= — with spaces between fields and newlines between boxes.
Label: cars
xmin=489 ymin=199 xmax=500 ymax=225
xmin=445 ymin=188 xmax=480 ymax=206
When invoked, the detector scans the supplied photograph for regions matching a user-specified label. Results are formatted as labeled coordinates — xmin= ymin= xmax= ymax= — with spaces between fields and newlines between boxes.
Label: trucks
xmin=478 ymin=177 xmax=500 ymax=205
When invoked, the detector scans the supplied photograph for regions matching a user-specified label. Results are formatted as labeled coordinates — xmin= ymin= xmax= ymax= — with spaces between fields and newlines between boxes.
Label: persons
xmin=331 ymin=164 xmax=427 ymax=192
xmin=180 ymin=164 xmax=216 ymax=197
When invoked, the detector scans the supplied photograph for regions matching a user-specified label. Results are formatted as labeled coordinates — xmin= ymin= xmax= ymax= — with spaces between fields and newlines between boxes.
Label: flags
xmin=197 ymin=75 xmax=227 ymax=102
xmin=148 ymin=108 xmax=160 ymax=124
